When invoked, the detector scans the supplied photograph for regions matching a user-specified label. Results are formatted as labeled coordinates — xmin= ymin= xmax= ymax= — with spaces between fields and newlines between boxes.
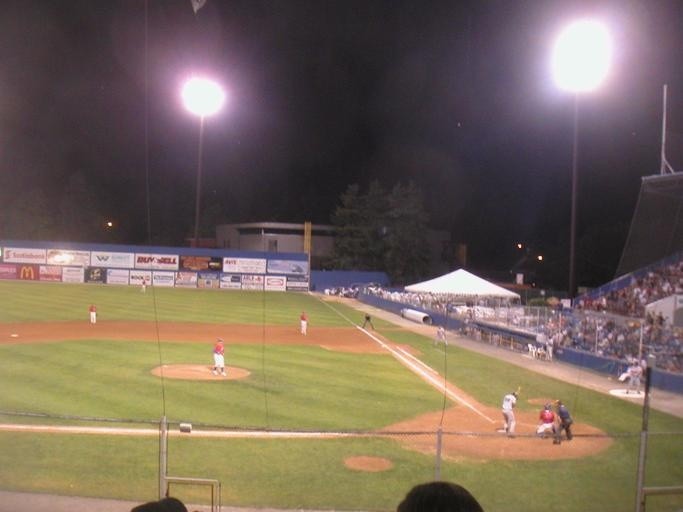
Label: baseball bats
xmin=517 ymin=386 xmax=520 ymax=396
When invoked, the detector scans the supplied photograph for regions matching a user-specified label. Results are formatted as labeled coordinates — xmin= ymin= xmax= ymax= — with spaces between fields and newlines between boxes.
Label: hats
xmin=555 ymin=400 xmax=561 ymax=404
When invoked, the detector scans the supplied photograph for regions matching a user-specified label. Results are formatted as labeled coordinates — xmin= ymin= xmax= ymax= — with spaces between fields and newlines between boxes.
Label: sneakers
xmin=212 ymin=370 xmax=226 ymax=376
xmin=553 ymin=440 xmax=560 ymax=444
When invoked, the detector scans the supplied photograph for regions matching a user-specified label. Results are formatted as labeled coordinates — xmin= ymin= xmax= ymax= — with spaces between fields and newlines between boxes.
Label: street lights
xmin=181 ymin=76 xmax=226 ymax=249
xmin=550 ymin=23 xmax=615 ymax=299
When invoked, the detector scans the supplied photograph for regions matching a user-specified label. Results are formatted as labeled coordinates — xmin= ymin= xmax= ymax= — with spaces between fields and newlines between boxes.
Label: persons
xmin=551 ymin=398 xmax=574 ymax=445
xmin=497 ymin=390 xmax=519 ymax=438
xmin=88 ymin=301 xmax=98 ymax=325
xmin=211 ymin=335 xmax=226 ymax=377
xmin=625 ymin=358 xmax=644 ymax=395
xmin=433 ymin=324 xmax=448 ymax=346
xmin=361 ymin=312 xmax=375 ymax=330
xmin=299 ymin=310 xmax=310 ymax=336
xmin=139 ymin=276 xmax=146 ymax=292
xmin=535 ymin=400 xmax=557 ymax=438
xmin=322 ymin=281 xmax=485 ymax=315
xmin=395 ymin=480 xmax=484 ymax=512
xmin=527 ymin=261 xmax=683 ymax=384
